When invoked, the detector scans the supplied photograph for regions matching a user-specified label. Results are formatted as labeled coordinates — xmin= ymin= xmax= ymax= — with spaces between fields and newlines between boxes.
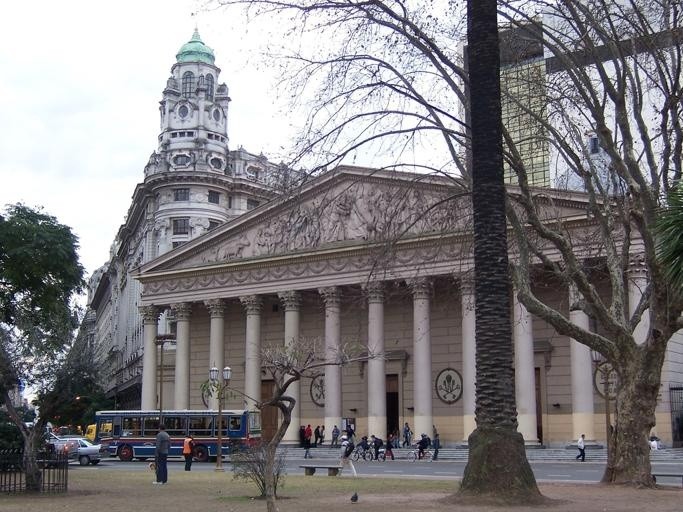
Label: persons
xmin=432 ymin=434 xmax=441 ymax=460
xmin=403 ymin=423 xmax=412 ymax=448
xmin=182 ymin=435 xmax=195 ymax=471
xmin=650 ymin=431 xmax=661 ymax=449
xmin=356 ymin=436 xmax=370 ymax=460
xmin=344 ymin=425 xmax=355 ymax=442
xmin=152 ymin=424 xmax=171 ymax=485
xmin=370 ymin=435 xmax=384 ymax=460
xmin=576 ymin=434 xmax=586 ymax=462
xmin=345 ymin=438 xmax=354 ymax=457
xmin=416 ymin=433 xmax=429 ymax=460
xmin=319 ymin=425 xmax=325 ymax=445
xmin=314 ymin=426 xmax=322 ymax=447
xmin=384 ymin=434 xmax=394 ymax=460
xmin=304 ymin=435 xmax=312 ymax=458
xmin=331 ymin=425 xmax=339 ymax=448
xmin=299 ymin=426 xmax=305 ymax=447
xmin=175 ymin=419 xmax=238 ymax=436
xmin=433 ymin=425 xmax=437 ymax=437
xmin=306 ymin=424 xmax=312 ymax=446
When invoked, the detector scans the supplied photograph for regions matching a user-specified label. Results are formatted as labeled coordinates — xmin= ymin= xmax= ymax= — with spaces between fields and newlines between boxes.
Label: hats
xmin=340 ymin=435 xmax=348 ymax=440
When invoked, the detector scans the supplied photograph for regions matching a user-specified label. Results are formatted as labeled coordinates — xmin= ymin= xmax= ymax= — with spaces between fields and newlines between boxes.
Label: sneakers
xmin=153 ymin=481 xmax=167 ymax=484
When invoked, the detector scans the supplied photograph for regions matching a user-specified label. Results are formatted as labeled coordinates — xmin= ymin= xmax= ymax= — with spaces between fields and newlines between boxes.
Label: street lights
xmin=589 ymin=347 xmax=614 ymax=459
xmin=75 ymin=383 xmax=117 ymax=410
xmin=209 ymin=361 xmax=232 ymax=472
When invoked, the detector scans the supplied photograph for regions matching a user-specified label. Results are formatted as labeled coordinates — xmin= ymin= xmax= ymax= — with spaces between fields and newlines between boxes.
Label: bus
xmin=84 ymin=410 xmax=263 ymax=462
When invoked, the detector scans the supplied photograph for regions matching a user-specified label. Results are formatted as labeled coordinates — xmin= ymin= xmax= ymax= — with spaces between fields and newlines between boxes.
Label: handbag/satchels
xmin=345 ymin=443 xmax=354 ymax=457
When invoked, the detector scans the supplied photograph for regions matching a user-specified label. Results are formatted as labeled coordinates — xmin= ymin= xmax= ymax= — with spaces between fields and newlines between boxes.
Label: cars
xmin=52 ymin=438 xmax=104 ymax=466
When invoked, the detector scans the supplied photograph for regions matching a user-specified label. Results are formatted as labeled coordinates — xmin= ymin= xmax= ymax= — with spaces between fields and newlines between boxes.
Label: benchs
xmin=298 ymin=465 xmax=343 ymax=476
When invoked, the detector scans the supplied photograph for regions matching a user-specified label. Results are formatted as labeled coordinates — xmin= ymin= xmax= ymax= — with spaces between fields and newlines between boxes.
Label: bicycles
xmin=406 ymin=445 xmax=433 ymax=463
xmin=350 ymin=443 xmax=386 ymax=463
